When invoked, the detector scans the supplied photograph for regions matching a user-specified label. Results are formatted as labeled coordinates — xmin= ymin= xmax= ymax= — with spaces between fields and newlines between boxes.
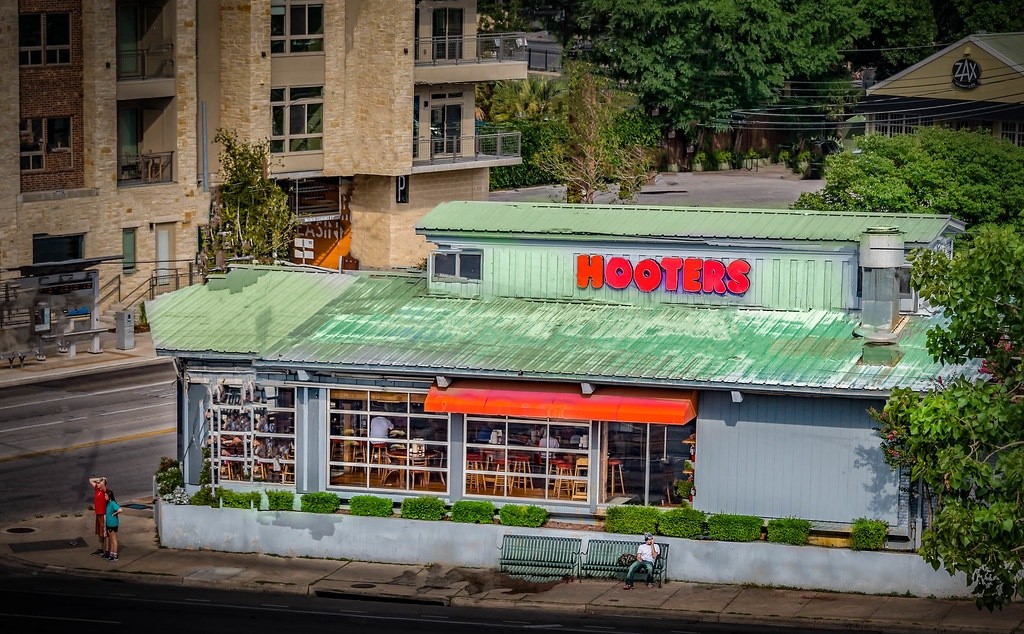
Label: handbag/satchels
xmin=617 ymin=553 xmax=636 ymax=567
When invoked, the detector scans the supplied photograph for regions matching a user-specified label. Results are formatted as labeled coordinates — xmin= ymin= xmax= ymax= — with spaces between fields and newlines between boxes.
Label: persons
xmin=88 ymin=477 xmax=111 ymax=559
xmin=369 ymin=409 xmax=396 ymax=448
xmin=478 ymin=422 xmax=496 ymax=440
xmin=103 ymin=490 xmax=123 ymax=561
xmin=622 ymin=534 xmax=661 ymax=589
xmin=539 ymin=429 xmax=560 ymax=463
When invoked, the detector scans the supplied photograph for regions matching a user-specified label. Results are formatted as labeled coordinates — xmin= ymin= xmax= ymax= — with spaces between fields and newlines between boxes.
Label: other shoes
xmin=101 ymin=551 xmax=110 ymax=559
xmin=623 ymin=584 xmax=634 ymax=589
xmin=91 ymin=548 xmax=104 ymax=556
xmin=113 ymin=553 xmax=119 ymax=561
xmin=648 ymin=583 xmax=653 ymax=588
xmin=107 ymin=552 xmax=114 ymax=560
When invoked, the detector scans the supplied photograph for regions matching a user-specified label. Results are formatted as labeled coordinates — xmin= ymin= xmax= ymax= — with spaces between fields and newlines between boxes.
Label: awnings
xmin=422 ymin=375 xmax=698 ymax=425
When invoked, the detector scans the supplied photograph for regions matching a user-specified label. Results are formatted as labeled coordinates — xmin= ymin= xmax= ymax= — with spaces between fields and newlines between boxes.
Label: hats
xmin=645 ymin=532 xmax=653 ymax=541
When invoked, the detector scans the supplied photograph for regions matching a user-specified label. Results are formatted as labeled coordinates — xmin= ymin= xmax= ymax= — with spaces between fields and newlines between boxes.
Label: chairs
xmin=409 ymin=458 xmax=429 ymax=490
xmin=571 ymin=457 xmax=588 ymax=501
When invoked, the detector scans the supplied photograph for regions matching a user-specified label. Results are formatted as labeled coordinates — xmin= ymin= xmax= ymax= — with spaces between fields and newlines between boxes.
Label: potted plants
xmin=717 ymin=152 xmax=733 ymax=169
xmin=692 ymin=152 xmax=707 ymax=171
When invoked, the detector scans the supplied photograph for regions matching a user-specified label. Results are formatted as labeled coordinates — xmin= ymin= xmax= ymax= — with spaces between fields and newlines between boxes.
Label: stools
xmin=0 ymin=351 xmax=18 ymax=370
xmin=16 ymin=347 xmax=40 ymax=369
xmin=221 ymin=443 xmax=627 ymax=502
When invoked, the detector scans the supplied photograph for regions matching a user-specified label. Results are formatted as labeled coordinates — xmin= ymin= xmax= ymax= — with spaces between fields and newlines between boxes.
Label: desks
xmin=386 ymin=448 xmax=438 ymax=488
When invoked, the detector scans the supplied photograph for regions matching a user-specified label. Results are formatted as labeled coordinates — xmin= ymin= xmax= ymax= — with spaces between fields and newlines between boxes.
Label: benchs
xmin=497 ymin=534 xmax=582 ymax=582
xmin=580 ymin=539 xmax=669 ymax=588
